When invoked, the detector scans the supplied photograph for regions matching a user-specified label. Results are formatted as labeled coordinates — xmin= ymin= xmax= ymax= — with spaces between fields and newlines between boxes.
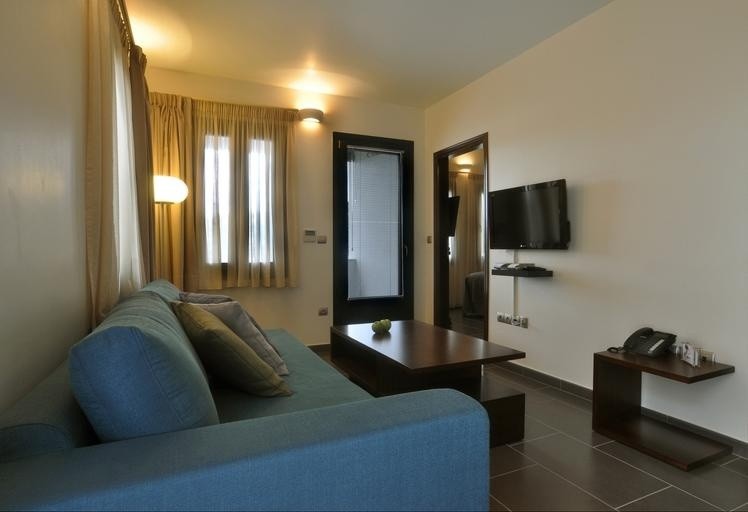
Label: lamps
xmin=296 ymin=107 xmax=325 ymax=124
xmin=152 ymin=174 xmax=190 ymax=281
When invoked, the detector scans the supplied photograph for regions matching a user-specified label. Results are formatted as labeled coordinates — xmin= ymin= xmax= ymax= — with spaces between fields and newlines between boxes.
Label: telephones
xmin=623 ymin=327 xmax=677 ymax=358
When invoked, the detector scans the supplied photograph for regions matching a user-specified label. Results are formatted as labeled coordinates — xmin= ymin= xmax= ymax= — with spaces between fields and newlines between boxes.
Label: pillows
xmin=177 ymin=289 xmax=283 ymax=360
xmin=193 ymin=297 xmax=291 ymax=379
xmin=163 ymin=298 xmax=293 ymax=398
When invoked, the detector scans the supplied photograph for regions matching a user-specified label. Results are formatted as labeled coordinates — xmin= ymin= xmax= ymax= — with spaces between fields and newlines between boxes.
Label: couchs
xmin=1 ymin=278 xmax=492 ymax=512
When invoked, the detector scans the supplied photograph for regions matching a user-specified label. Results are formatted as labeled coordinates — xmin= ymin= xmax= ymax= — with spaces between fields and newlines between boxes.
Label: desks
xmin=587 ymin=345 xmax=737 ymax=475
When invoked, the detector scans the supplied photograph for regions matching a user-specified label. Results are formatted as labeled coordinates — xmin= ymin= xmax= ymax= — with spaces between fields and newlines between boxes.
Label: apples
xmin=372 ymin=319 xmax=391 ymax=333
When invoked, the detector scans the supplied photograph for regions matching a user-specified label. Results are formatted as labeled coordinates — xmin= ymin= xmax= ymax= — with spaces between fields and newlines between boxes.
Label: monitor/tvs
xmin=488 ymin=179 xmax=569 ymax=249
xmin=449 ymin=195 xmax=460 ymax=237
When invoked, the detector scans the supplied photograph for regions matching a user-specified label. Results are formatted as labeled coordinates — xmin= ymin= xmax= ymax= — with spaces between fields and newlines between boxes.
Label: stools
xmin=459 ymin=272 xmax=485 ymax=318
